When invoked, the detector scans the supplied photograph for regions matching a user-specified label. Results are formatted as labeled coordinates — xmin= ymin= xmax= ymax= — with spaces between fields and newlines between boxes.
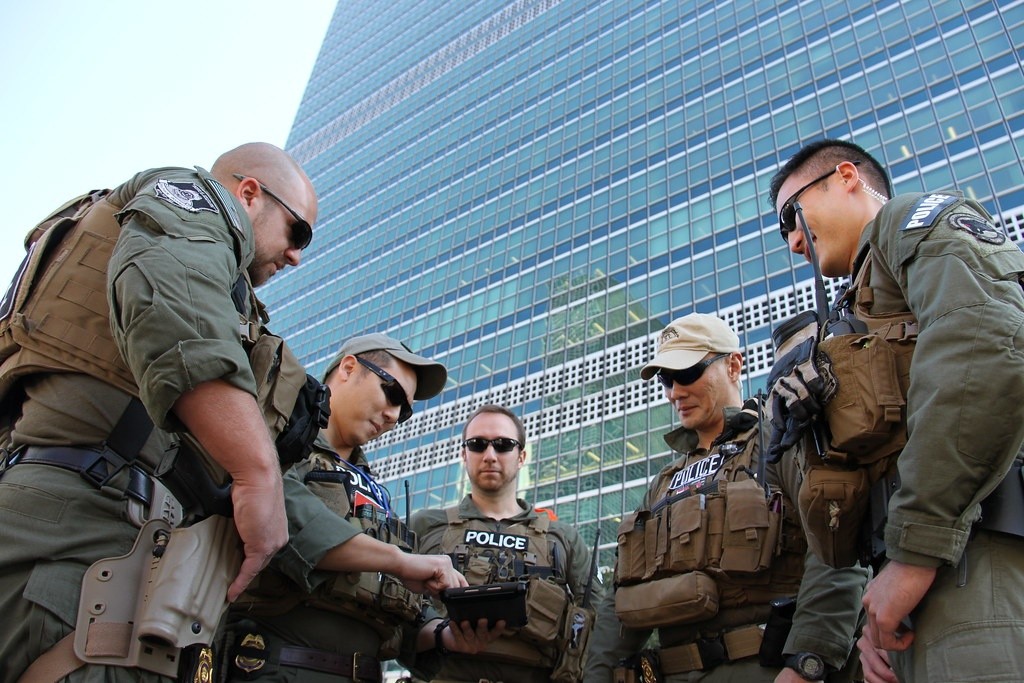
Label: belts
xmin=278 ymin=644 xmax=383 ymax=683
xmin=6 ymin=445 xmax=154 ymax=507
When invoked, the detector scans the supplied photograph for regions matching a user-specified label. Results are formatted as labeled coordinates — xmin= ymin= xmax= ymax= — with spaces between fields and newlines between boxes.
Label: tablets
xmin=440 ymin=582 xmax=528 ymax=629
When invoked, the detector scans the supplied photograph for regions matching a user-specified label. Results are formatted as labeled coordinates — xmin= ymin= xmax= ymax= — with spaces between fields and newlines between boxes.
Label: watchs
xmin=784 ymin=651 xmax=828 ymax=680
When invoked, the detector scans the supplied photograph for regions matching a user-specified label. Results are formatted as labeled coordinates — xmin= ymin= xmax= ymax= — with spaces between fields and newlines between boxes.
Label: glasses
xmin=657 ymin=354 xmax=731 ymax=386
xmin=779 ymin=160 xmax=861 ymax=244
xmin=463 ymin=437 xmax=523 ymax=452
xmin=232 ymin=173 xmax=312 ymax=249
xmin=356 ymin=356 xmax=413 ymax=423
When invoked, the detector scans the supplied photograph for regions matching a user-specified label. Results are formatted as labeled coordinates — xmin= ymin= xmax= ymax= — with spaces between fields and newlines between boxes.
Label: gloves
xmin=766 ymin=310 xmax=825 ymax=464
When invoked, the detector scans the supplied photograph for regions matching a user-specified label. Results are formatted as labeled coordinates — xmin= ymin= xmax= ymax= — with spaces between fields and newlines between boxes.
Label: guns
xmin=139 ymin=440 xmax=233 ymax=651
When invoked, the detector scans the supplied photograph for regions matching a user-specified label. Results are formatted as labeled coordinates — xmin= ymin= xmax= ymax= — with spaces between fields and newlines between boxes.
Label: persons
xmin=0 ymin=142 xmax=318 ymax=683
xmin=212 ymin=332 xmax=505 ymax=683
xmin=585 ymin=313 xmax=871 ymax=683
xmin=769 ymin=139 xmax=1024 ymax=683
xmin=409 ymin=404 xmax=607 ymax=683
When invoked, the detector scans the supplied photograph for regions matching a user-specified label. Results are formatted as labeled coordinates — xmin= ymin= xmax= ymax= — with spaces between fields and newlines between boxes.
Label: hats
xmin=322 ymin=333 xmax=448 ymax=400
xmin=640 ymin=311 xmax=740 ymax=380
